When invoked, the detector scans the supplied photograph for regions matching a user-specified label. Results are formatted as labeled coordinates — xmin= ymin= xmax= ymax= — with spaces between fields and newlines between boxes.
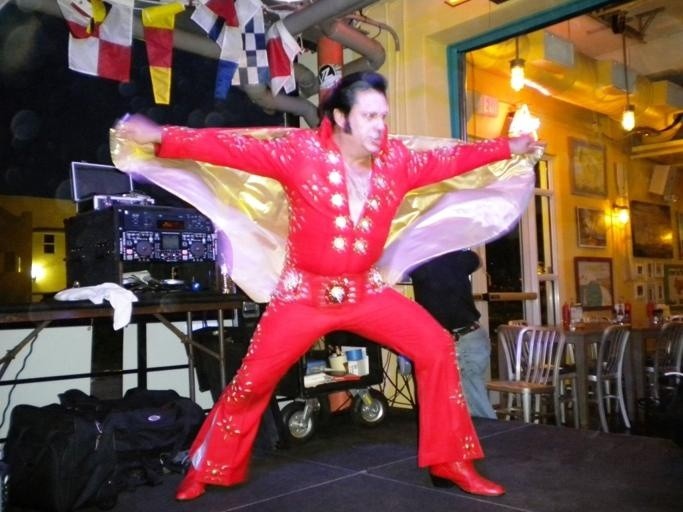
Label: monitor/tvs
xmin=69 ymin=161 xmax=135 ymax=203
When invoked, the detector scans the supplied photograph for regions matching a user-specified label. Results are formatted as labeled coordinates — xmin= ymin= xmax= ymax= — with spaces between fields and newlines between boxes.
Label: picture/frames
xmin=576 ymin=208 xmax=610 ymax=249
xmin=574 ymin=257 xmax=615 ymax=311
xmin=567 ymin=135 xmax=608 ymax=200
xmin=664 ymin=264 xmax=683 ymax=313
xmin=630 ymin=200 xmax=674 ymax=259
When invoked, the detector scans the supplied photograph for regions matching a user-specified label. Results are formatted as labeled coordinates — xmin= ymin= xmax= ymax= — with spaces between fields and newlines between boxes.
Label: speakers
xmin=61 ymin=205 xmax=216 ymax=296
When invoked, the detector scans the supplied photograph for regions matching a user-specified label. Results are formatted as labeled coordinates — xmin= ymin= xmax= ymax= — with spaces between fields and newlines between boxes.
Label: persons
xmin=409 ymin=247 xmax=498 ymax=421
xmin=116 ymin=69 xmax=548 ymax=500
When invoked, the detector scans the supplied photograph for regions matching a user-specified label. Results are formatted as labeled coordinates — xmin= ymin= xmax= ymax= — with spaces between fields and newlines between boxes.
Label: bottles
xmin=563 ymin=300 xmax=583 ymax=332
xmin=346 ymin=349 xmax=365 ymax=375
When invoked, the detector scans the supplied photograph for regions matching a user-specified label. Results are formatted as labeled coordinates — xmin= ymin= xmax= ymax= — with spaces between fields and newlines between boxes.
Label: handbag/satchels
xmin=1 ymin=388 xmax=206 ymax=512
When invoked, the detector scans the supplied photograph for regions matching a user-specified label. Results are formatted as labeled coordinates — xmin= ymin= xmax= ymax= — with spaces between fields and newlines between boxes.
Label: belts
xmin=458 ymin=324 xmax=479 ymax=335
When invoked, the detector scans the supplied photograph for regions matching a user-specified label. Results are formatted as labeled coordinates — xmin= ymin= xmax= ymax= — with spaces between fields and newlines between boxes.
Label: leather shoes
xmin=429 ymin=457 xmax=505 ymax=497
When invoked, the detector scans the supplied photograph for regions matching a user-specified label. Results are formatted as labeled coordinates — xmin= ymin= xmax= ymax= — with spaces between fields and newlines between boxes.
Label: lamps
xmin=508 ymin=37 xmax=526 ymax=92
xmin=620 ymin=34 xmax=636 ymax=132
xmin=615 ymin=196 xmax=630 ymax=224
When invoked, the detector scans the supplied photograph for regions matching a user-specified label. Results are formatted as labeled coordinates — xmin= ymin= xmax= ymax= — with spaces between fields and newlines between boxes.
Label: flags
xmin=66 ymin=0 xmax=134 ymax=84
xmin=58 ymin=0 xmax=113 ymax=39
xmin=142 ymin=0 xmax=304 ymax=105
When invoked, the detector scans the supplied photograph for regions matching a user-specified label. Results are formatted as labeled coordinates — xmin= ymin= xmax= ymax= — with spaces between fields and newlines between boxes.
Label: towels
xmin=54 ymin=282 xmax=139 ymax=332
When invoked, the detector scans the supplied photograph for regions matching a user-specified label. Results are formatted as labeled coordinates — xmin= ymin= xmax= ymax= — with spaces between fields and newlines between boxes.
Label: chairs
xmin=483 ymin=314 xmax=683 ymax=434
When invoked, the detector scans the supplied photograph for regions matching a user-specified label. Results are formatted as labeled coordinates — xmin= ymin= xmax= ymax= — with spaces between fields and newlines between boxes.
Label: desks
xmin=0 ymin=292 xmax=255 ymax=407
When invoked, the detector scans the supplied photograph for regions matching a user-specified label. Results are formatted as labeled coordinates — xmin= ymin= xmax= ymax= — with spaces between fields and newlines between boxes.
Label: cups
xmin=328 ymin=354 xmax=348 ymax=373
